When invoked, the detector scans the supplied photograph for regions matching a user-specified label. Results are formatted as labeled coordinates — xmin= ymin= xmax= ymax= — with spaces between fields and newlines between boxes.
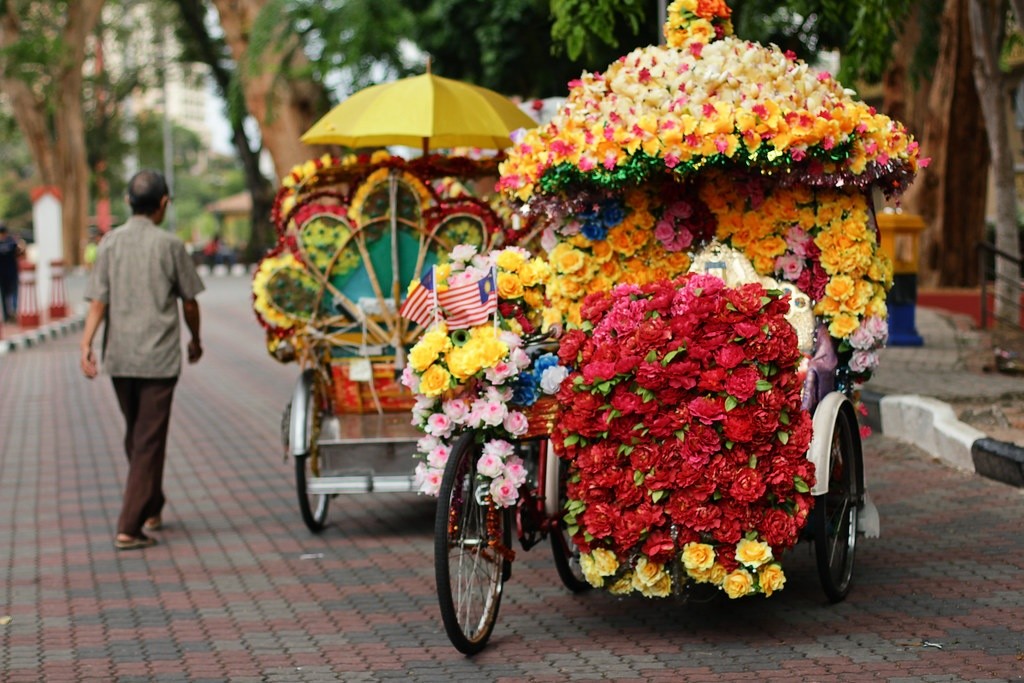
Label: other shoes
xmin=145 ymin=518 xmax=160 ymax=531
xmin=116 ymin=535 xmax=156 ymax=549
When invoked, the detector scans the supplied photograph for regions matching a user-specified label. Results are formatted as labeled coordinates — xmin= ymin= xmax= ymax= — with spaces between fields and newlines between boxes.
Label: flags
xmin=400 ymin=264 xmax=497 ymax=330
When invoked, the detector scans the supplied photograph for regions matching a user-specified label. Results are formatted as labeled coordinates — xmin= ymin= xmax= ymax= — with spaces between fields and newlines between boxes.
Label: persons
xmin=0 ymin=223 xmax=20 ymax=321
xmin=205 ymin=233 xmax=235 ymax=274
xmin=79 ymin=169 xmax=204 ymax=550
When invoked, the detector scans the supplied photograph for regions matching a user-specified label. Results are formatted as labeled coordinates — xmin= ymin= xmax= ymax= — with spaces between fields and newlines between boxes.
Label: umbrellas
xmin=299 ymin=56 xmax=538 ymax=183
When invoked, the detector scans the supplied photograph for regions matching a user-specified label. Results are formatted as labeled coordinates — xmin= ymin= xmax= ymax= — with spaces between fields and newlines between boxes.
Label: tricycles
xmin=290 ymin=55 xmax=482 ymax=534
xmin=433 ymin=323 xmax=867 ymax=658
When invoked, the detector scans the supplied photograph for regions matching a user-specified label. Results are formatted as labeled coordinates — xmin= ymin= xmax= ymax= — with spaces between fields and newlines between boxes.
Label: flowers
xmin=252 ymin=0 xmax=933 ymax=598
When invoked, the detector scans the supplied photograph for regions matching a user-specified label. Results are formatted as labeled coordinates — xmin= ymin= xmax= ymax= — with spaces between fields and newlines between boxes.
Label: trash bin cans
xmin=886 ymin=270 xmax=925 ymax=347
